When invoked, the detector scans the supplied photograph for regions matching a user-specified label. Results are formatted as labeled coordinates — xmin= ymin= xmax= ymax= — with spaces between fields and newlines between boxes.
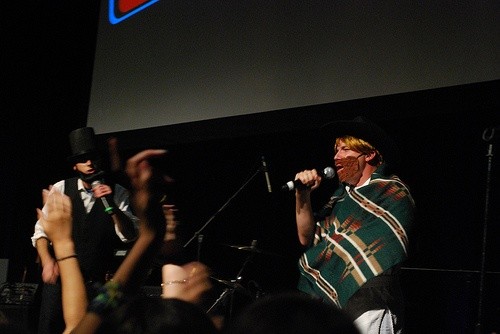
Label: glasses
xmin=73 ymin=153 xmax=99 ymax=163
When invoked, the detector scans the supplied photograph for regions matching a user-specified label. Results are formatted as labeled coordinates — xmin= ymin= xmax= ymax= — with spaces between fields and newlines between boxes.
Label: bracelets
xmin=54 ymin=255 xmax=79 ymax=261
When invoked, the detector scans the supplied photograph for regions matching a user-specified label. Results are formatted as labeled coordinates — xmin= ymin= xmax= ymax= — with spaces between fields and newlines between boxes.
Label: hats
xmin=320 ymin=114 xmax=403 ymax=172
xmin=68 ymin=126 xmax=105 ymax=160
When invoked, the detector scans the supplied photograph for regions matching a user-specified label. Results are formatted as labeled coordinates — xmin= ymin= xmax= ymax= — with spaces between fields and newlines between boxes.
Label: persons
xmin=30 ymin=126 xmax=360 ymax=334
xmin=293 ymin=117 xmax=417 ymax=334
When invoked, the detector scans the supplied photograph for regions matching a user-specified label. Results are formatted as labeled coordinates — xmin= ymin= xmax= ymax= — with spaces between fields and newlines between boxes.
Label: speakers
xmin=0 ymin=282 xmax=41 ymax=334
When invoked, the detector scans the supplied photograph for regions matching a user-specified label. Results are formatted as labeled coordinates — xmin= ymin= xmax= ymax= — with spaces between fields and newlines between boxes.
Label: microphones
xmin=282 ymin=167 xmax=335 ymax=191
xmin=262 ymin=157 xmax=272 ymax=193
xmin=91 ymin=181 xmax=113 ymax=216
